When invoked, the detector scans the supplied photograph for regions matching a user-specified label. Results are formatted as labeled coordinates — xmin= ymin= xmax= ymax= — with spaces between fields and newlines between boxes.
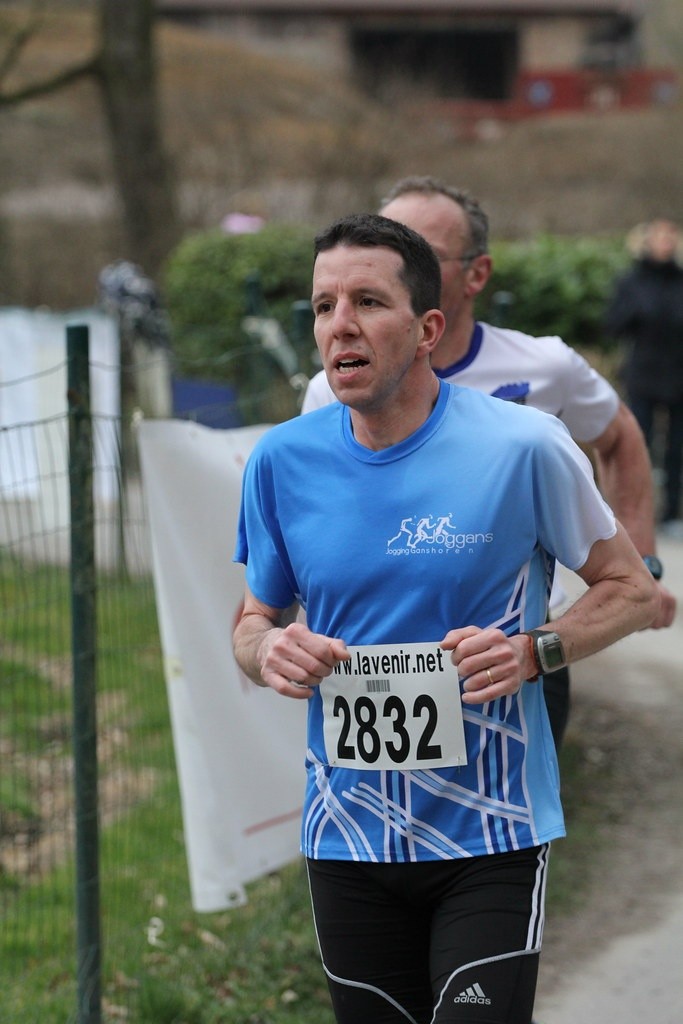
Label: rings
xmin=486 ymin=668 xmax=493 ymax=685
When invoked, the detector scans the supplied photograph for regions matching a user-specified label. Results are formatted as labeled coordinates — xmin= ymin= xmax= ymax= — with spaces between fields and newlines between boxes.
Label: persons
xmin=234 ymin=177 xmax=683 ymax=754
xmin=234 ymin=213 xmax=663 ymax=1024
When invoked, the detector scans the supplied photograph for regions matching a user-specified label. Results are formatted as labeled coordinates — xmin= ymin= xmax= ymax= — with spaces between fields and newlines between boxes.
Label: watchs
xmin=519 ymin=629 xmax=567 ymax=683
xmin=642 ymin=555 xmax=663 ymax=581
